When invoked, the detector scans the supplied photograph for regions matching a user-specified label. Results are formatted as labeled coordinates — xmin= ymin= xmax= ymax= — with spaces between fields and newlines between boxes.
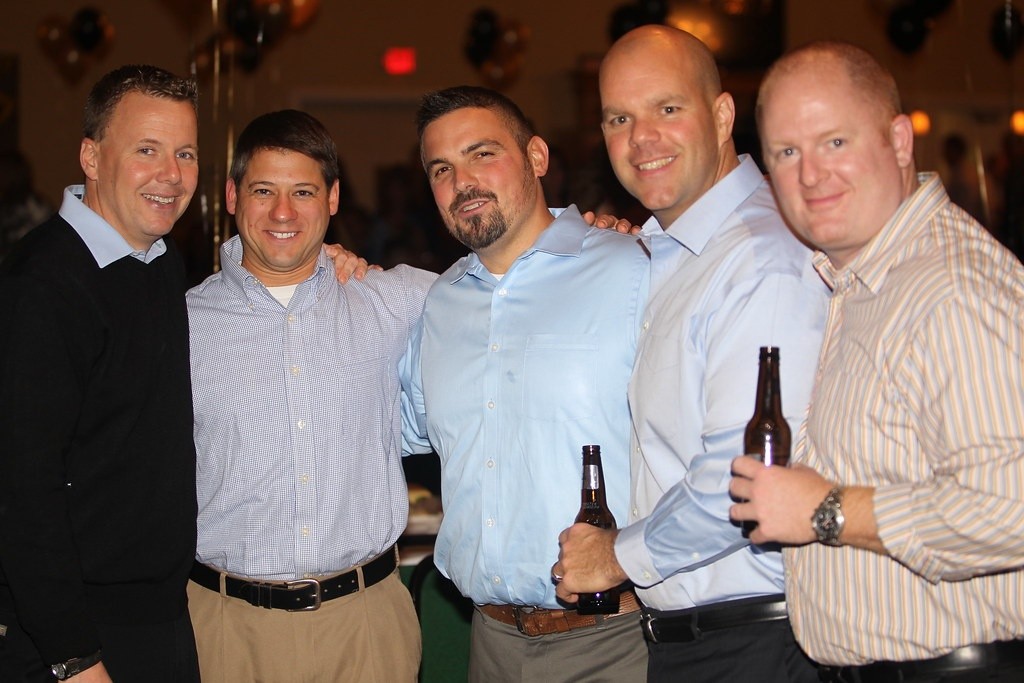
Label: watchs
xmin=812 ymin=488 xmax=843 ymax=546
xmin=51 ymin=650 xmax=102 ymax=680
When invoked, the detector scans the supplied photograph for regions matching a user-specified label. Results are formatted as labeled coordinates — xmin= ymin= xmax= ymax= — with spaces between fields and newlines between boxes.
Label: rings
xmin=552 ymin=562 xmax=562 ymax=580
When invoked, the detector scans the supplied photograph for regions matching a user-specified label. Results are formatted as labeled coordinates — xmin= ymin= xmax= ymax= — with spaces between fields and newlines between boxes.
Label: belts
xmin=817 ymin=639 xmax=1016 ymax=683
xmin=479 ymin=588 xmax=643 ymax=636
xmin=637 ymin=598 xmax=788 ymax=644
xmin=188 ymin=542 xmax=397 ymax=612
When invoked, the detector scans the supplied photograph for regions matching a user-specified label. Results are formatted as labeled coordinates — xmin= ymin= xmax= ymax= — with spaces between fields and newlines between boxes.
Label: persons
xmin=185 ymin=108 xmax=643 ymax=683
xmin=727 ymin=40 xmax=1024 ymax=683
xmin=551 ymin=22 xmax=830 ymax=683
xmin=0 ymin=62 xmax=385 ymax=683
xmin=397 ymin=86 xmax=651 ymax=683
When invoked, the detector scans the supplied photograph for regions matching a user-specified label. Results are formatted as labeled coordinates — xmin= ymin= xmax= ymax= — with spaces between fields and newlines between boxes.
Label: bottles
xmin=577 ymin=445 xmax=620 ymax=615
xmin=742 ymin=347 xmax=792 ymax=539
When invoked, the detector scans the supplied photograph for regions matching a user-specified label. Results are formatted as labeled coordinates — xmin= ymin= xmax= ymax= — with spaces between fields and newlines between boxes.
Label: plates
xmin=404 ymin=515 xmax=443 ymax=534
